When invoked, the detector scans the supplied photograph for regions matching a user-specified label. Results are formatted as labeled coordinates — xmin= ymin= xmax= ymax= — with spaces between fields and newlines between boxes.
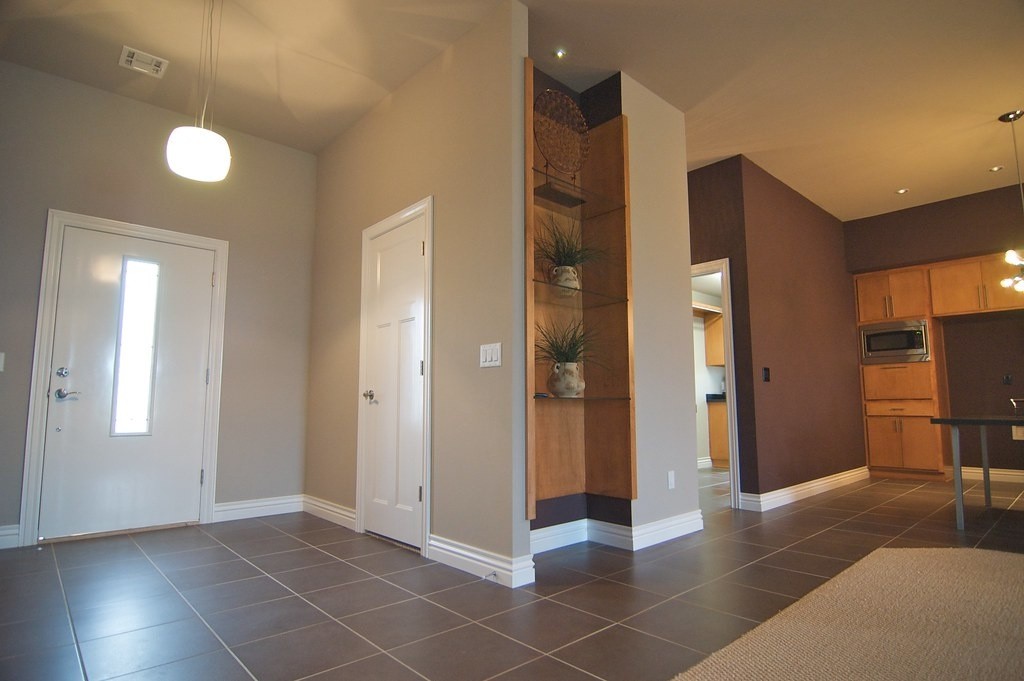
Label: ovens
xmin=858 ymin=316 xmax=931 ymax=365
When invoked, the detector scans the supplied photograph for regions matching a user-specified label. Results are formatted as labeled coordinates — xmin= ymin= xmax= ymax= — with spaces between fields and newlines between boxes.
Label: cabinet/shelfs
xmin=857 ymin=315 xmax=954 ymax=484
xmin=531 ymin=168 xmax=629 ymax=398
xmin=853 ymin=248 xmax=1024 ymax=327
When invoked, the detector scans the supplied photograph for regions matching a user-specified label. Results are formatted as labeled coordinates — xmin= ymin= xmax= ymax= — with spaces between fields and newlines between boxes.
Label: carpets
xmin=668 ymin=542 xmax=1024 ymax=681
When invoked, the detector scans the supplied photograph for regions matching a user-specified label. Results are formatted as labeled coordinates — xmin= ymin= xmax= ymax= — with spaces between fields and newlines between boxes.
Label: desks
xmin=931 ymin=412 xmax=1024 ymax=530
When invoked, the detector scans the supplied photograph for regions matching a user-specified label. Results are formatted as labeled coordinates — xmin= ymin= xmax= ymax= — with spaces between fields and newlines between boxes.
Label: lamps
xmin=165 ymin=0 xmax=231 ymax=184
xmin=996 ymin=109 xmax=1024 ymax=294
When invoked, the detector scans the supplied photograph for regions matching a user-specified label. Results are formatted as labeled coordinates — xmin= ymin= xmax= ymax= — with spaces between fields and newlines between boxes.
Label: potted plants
xmin=533 ymin=207 xmax=610 ymax=297
xmin=534 ymin=312 xmax=613 ymax=397
